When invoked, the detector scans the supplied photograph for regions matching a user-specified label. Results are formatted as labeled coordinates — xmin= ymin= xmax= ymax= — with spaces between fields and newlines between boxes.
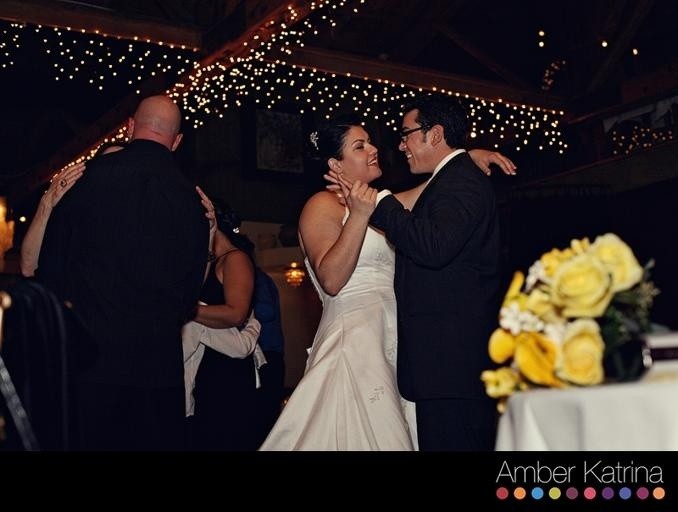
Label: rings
xmin=60 ymin=179 xmax=68 ymax=188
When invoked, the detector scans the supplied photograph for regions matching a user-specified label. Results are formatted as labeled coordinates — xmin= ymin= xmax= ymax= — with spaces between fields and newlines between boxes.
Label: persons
xmin=322 ymin=95 xmax=498 ymax=452
xmin=253 ymin=265 xmax=284 ymax=419
xmin=34 ymin=95 xmax=210 ymax=453
xmin=18 ymin=141 xmax=217 ymax=293
xmin=198 ymin=198 xmax=256 ymax=450
xmin=257 ymin=122 xmax=520 ymax=452
xmin=181 ymin=302 xmax=264 ymax=420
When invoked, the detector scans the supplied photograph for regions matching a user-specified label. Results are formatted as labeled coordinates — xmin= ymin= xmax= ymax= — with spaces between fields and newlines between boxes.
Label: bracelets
xmin=207 ymin=251 xmax=216 ymax=262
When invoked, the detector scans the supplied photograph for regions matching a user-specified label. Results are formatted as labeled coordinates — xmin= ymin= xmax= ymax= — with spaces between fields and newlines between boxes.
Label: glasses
xmin=398 ymin=122 xmax=436 ymax=143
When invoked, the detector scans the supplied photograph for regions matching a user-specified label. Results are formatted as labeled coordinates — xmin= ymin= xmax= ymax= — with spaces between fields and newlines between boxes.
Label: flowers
xmin=480 ymin=233 xmax=660 ymax=413
